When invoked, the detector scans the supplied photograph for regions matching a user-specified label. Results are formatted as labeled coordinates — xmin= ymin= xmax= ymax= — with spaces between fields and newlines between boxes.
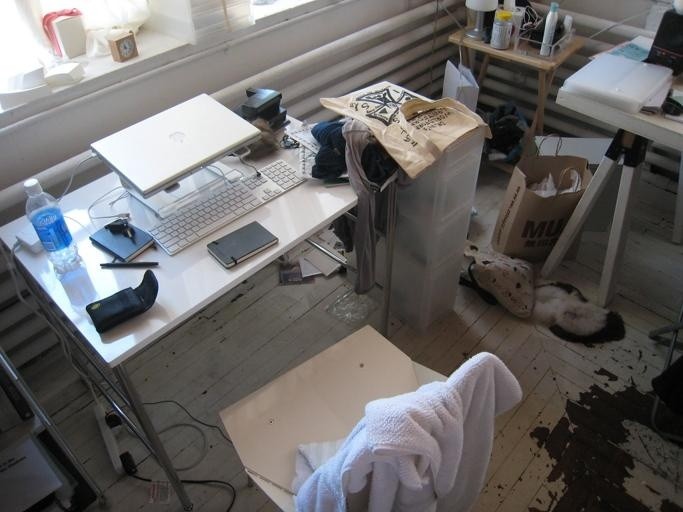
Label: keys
xmin=120 ymin=226 xmax=137 ymax=246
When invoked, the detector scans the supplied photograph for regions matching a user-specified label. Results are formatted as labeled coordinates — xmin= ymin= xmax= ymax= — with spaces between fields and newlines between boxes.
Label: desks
xmin=446 ymin=22 xmax=589 ymax=175
xmin=541 ymin=34 xmax=682 ymax=309
xmin=1 ymin=103 xmax=360 ymax=512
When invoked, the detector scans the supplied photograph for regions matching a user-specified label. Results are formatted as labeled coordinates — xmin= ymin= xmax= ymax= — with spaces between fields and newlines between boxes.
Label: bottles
xmin=24 ymin=178 xmax=79 ymax=266
xmin=490 ymin=9 xmax=513 ymax=50
xmin=539 ymin=2 xmax=558 ymax=57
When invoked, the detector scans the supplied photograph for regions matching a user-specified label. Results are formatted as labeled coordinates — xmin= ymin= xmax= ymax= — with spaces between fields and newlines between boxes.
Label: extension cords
xmin=92 ymin=403 xmax=125 ymax=475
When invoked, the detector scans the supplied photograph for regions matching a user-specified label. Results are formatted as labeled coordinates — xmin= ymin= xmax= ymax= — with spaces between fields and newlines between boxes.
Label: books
xmin=281 ymin=121 xmax=350 ymax=187
xmin=88 ymin=220 xmax=155 ymax=264
xmin=205 ymin=221 xmax=279 ymax=269
xmin=641 ymin=82 xmax=674 ymax=116
xmin=664 ymin=89 xmax=683 ymax=124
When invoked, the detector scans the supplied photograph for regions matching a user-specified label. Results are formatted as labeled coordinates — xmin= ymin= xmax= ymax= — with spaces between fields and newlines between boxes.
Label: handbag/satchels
xmin=482 ymin=104 xmax=537 ymax=166
xmin=489 ymin=154 xmax=594 ymax=265
xmin=319 ymin=81 xmax=494 ymax=180
xmin=459 ymin=240 xmax=534 ymax=319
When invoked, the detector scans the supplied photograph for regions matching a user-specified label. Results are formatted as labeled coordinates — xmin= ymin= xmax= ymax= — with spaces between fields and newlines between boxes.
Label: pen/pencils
xmin=100 ymin=263 xmax=158 ymax=267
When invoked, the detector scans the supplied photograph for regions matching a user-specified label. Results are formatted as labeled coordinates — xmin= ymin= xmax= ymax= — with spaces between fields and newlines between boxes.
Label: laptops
xmin=89 ymin=93 xmax=262 ymax=199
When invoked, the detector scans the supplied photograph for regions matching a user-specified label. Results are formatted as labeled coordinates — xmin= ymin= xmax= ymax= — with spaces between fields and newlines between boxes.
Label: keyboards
xmin=144 ymin=159 xmax=307 ymax=257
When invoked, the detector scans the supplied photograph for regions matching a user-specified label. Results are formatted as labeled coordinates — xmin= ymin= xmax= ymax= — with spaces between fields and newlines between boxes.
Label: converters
xmin=15 ymin=223 xmax=44 ymax=254
xmin=119 ymin=451 xmax=137 ymax=476
xmin=106 ymin=414 xmax=123 ymax=429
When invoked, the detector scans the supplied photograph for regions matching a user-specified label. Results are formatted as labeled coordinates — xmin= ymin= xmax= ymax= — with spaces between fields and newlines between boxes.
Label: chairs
xmin=218 ymin=321 xmax=500 ymax=512
